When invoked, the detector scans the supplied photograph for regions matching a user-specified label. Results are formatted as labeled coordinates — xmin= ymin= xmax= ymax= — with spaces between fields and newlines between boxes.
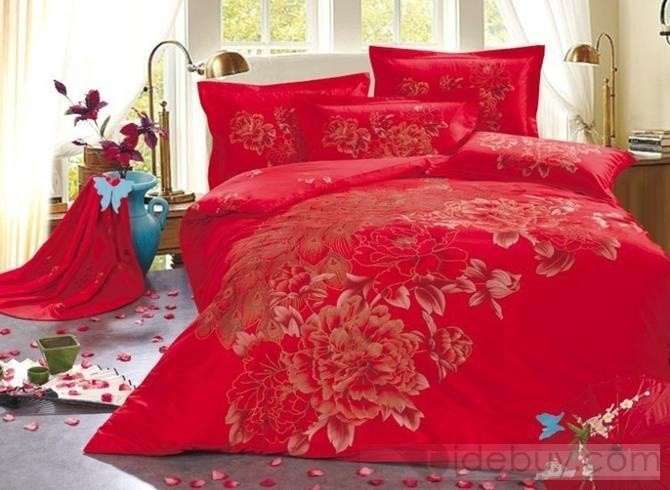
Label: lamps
xmin=563 ymin=30 xmax=618 ymax=148
xmin=144 ymin=40 xmax=250 ymax=204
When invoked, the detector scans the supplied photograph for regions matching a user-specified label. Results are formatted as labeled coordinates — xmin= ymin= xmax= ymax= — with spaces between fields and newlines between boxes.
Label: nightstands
xmin=612 ymin=148 xmax=668 ymax=251
xmin=146 ymin=199 xmax=200 ymax=270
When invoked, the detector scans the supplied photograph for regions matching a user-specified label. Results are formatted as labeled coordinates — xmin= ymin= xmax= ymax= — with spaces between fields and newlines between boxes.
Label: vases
xmin=97 ymin=169 xmax=168 ymax=278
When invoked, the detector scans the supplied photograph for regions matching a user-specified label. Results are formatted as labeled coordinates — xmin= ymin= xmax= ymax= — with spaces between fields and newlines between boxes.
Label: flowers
xmin=52 ymin=78 xmax=171 ymax=180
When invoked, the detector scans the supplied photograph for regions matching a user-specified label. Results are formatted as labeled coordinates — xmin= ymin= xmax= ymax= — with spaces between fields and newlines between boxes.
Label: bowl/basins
xmin=27 ymin=366 xmax=49 ymax=385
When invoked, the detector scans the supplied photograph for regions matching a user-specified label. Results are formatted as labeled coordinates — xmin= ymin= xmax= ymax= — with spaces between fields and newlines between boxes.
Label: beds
xmin=206 ymin=53 xmax=670 ymax=457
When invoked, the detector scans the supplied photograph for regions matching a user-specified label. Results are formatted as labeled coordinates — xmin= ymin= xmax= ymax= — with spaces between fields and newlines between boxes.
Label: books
xmin=143 ymin=192 xmax=194 ymax=204
xmin=48 ymin=203 xmax=67 ymax=213
xmin=627 ymin=128 xmax=669 ymax=160
xmin=50 ymin=195 xmax=61 ymax=204
